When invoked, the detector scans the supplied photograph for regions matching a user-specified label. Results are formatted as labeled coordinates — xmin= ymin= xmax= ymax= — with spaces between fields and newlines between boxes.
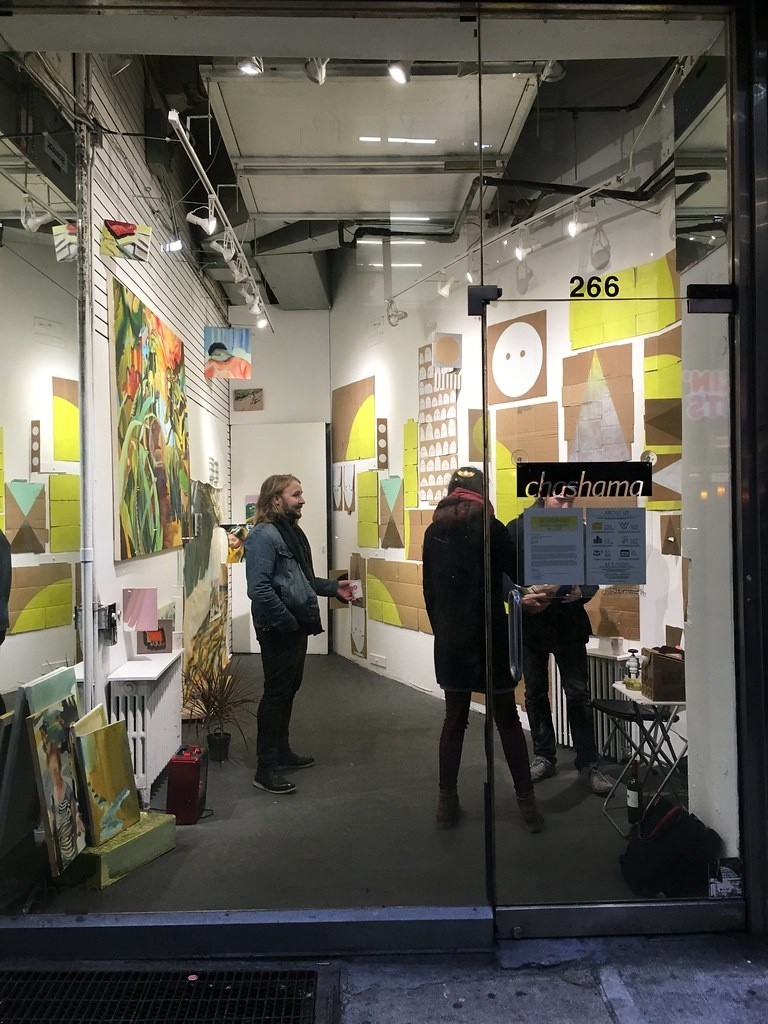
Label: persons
xmin=244 ymin=474 xmax=364 ymax=794
xmin=422 ymin=466 xmax=545 ymax=833
xmin=46 ymin=744 xmax=78 ymax=875
xmin=506 ymin=497 xmax=614 ymax=797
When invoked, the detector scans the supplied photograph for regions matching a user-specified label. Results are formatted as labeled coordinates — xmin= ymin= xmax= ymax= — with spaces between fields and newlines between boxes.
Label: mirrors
xmin=0 ymin=50 xmax=84 ymax=891
xmin=671 ymin=14 xmax=730 ymax=274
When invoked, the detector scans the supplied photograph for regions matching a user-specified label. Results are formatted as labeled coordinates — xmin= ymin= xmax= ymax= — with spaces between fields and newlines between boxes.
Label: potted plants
xmin=180 ymin=636 xmax=261 ymax=769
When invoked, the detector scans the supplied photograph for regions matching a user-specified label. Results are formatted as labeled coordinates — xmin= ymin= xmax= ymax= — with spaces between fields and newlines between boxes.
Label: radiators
xmin=108 ymin=652 xmax=183 ymax=812
xmin=549 ymin=648 xmax=639 ymax=766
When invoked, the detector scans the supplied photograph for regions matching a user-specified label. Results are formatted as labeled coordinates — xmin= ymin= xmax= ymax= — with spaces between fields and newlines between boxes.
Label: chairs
xmin=590 ymin=698 xmax=682 ymax=790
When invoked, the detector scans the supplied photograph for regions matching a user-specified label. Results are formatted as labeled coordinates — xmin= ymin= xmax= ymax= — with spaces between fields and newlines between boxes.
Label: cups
xmin=348 ymin=579 xmax=363 ymax=599
xmin=610 ymin=636 xmax=624 ymax=656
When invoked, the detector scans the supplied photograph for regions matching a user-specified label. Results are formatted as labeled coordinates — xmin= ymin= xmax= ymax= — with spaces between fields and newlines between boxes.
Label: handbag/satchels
xmin=618 ymin=794 xmax=725 ymax=898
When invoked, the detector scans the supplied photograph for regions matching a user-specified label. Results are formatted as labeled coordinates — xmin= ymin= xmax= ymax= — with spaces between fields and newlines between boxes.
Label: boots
xmin=516 ymin=794 xmax=544 ymax=834
xmin=436 ymin=792 xmax=460 ymax=821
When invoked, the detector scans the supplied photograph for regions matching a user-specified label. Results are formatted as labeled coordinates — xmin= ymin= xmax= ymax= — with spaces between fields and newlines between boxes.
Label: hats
xmin=447 ymin=466 xmax=484 ymax=494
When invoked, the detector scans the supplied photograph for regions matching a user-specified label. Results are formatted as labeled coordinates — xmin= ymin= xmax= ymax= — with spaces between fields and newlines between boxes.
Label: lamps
xmin=238 ymin=278 xmax=255 ymax=303
xmin=256 ymin=315 xmax=267 ymax=329
xmin=248 ymin=296 xmax=262 ymax=314
xmin=466 ymin=253 xmax=490 ymax=284
xmin=105 ymin=54 xmax=132 ymax=77
xmin=541 ymin=60 xmax=567 ymax=83
xmin=302 ymin=57 xmax=332 ymax=86
xmin=437 ymin=268 xmax=456 ymax=299
xmin=210 ymin=226 xmax=235 ymax=263
xmin=388 ymin=60 xmax=414 ymax=85
xmin=21 ymin=194 xmax=56 ymax=233
xmin=227 ymin=253 xmax=248 ymax=285
xmin=386 ymin=300 xmax=408 ymax=328
xmin=185 ymin=198 xmax=217 ymax=235
xmin=457 ymin=61 xmax=479 ymax=78
xmin=568 ymin=198 xmax=599 ymax=240
xmin=236 ymin=56 xmax=265 ymax=75
xmin=514 ymin=224 xmax=542 ymax=263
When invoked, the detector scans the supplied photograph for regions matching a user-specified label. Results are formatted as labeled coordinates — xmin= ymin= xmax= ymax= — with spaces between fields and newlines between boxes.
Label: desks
xmin=603 ymin=681 xmax=688 ymax=839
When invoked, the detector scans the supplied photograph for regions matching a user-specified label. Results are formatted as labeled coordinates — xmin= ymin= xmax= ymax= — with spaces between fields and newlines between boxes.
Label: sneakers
xmin=530 ymin=755 xmax=558 ymax=781
xmin=589 ymin=767 xmax=614 ymax=794
xmin=253 ymin=770 xmax=296 ymax=793
xmin=272 ymin=750 xmax=318 ymax=769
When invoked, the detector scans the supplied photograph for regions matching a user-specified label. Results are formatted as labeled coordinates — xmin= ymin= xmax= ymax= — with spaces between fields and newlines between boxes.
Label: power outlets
xmin=369 ymin=653 xmax=386 ymax=668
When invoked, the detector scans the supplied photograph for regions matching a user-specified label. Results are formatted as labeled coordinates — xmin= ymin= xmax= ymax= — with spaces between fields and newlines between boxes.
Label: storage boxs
xmin=46 ymin=810 xmax=176 ymax=890
xmin=665 ymin=624 xmax=685 ymax=650
xmin=610 ymin=636 xmax=625 ymax=655
xmin=641 ymin=648 xmax=685 ymax=702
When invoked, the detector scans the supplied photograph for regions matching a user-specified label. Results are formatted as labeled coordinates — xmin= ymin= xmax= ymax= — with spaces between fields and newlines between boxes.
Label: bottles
xmin=626 ymin=760 xmax=644 ymax=824
